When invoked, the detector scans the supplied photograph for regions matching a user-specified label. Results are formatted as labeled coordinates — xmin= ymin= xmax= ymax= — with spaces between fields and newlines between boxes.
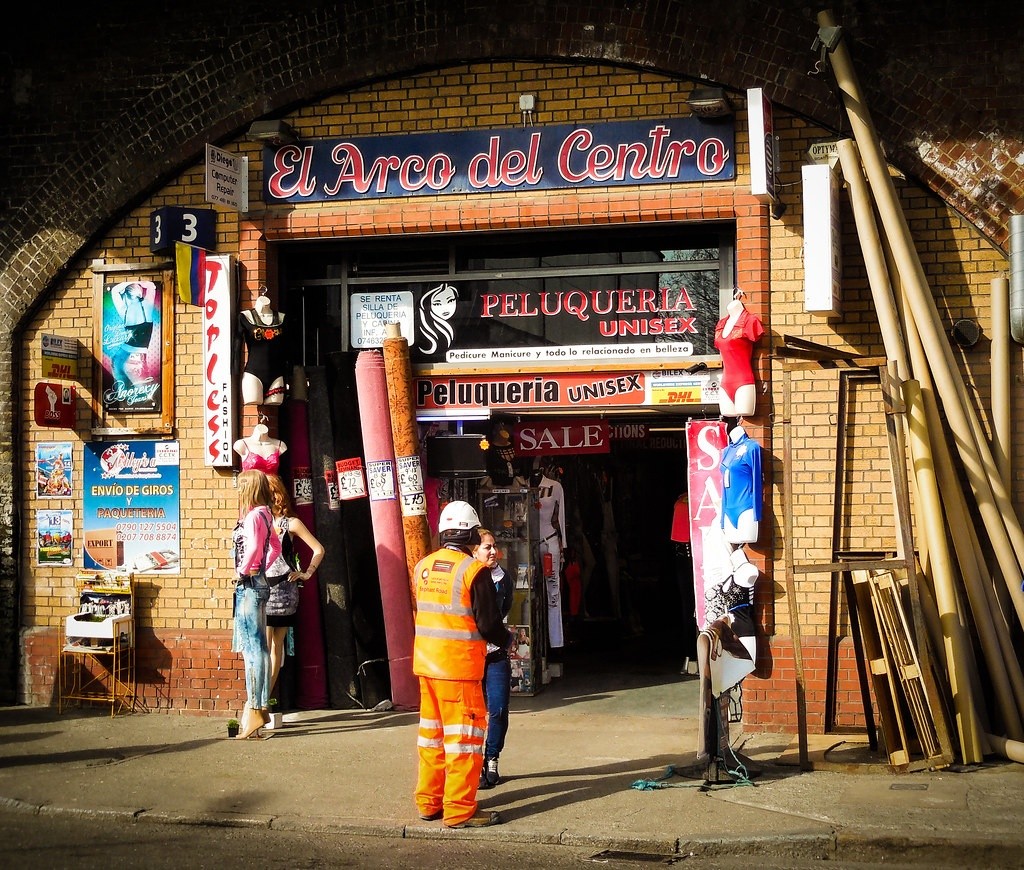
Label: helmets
xmin=438 ymin=502 xmax=481 ymax=535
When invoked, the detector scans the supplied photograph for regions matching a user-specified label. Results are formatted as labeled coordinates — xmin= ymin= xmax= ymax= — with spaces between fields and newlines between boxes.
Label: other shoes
xmin=418 ymin=809 xmax=443 ymax=821
xmin=447 ymin=810 xmax=501 ymax=829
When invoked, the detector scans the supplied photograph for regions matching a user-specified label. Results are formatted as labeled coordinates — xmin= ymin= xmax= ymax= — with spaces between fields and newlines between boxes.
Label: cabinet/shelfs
xmin=477 ymin=487 xmax=548 ymax=696
xmin=57 ymin=569 xmax=135 ymax=719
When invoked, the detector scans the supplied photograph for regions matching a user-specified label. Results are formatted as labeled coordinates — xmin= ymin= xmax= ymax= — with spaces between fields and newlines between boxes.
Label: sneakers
xmin=477 ymin=756 xmax=500 ymax=790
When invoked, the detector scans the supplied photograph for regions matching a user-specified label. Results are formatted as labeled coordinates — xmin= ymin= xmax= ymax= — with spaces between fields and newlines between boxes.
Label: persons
xmin=671 ymin=493 xmax=700 ymax=675
xmin=507 ymin=628 xmax=531 ymax=692
xmin=232 ymin=422 xmax=287 ymax=481
xmin=519 ymin=471 xmax=568 ymax=648
xmin=489 ymin=428 xmax=520 ymax=486
xmin=110 ymin=280 xmax=156 ymax=400
xmin=720 ymin=425 xmax=762 ymax=543
xmin=230 ymin=469 xmax=326 ymax=740
xmin=714 ymin=300 xmax=764 ymax=418
xmin=237 ymin=296 xmax=291 ymax=405
xmin=719 ymin=548 xmax=758 ymax=671
xmin=413 ymin=501 xmax=519 ymax=830
